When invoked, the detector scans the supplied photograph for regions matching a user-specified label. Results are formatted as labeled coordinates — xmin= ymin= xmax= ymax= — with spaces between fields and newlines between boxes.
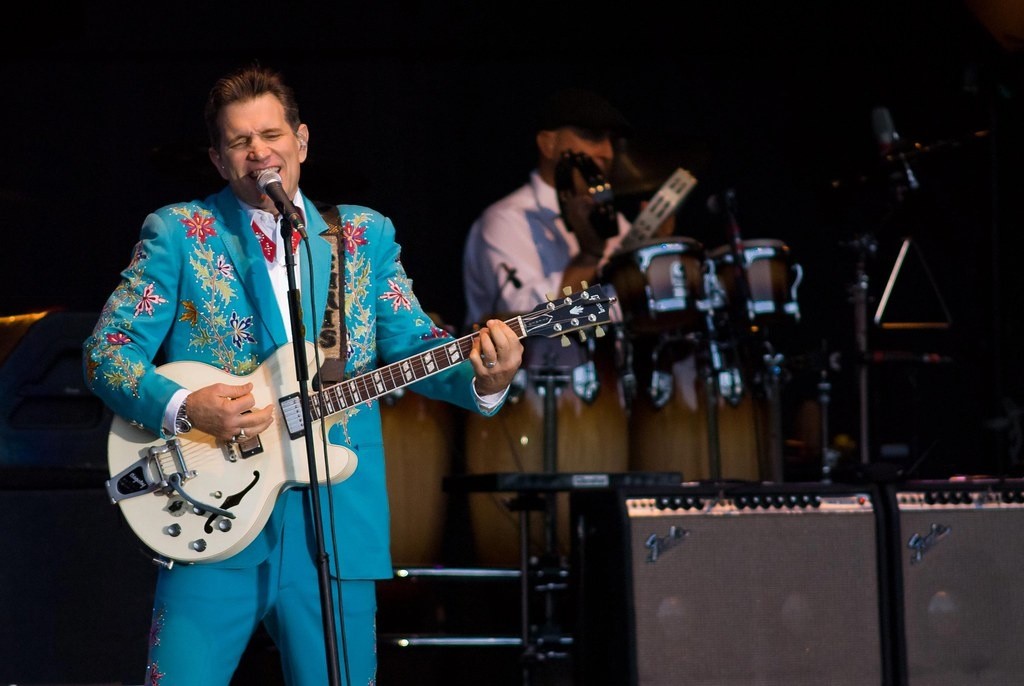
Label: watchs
xmin=176 ymin=397 xmax=193 ymax=434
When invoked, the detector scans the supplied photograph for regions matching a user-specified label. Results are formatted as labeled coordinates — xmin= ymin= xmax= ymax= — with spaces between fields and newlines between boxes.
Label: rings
xmin=237 ymin=428 xmax=247 ymax=441
xmin=226 ymin=436 xmax=236 ymax=444
xmin=483 ymin=359 xmax=497 ymax=368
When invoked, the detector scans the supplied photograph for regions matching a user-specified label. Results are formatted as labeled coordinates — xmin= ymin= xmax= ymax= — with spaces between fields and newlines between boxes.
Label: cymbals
xmin=553 ymin=147 xmax=620 ymax=238
xmin=620 ymin=167 xmax=700 ymax=248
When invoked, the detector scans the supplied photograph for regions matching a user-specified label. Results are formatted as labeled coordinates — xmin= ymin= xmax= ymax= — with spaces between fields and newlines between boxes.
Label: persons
xmin=462 ymin=95 xmax=675 ymax=409
xmin=77 ymin=72 xmax=523 ymax=686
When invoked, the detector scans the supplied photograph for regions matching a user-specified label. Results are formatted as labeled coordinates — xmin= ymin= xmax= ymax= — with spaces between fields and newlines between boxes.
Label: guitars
xmin=105 ymin=280 xmax=619 ymax=569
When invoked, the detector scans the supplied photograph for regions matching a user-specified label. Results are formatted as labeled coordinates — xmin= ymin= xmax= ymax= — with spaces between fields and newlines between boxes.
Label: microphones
xmin=865 ymin=101 xmax=920 ymax=197
xmin=256 ymin=170 xmax=310 ymax=245
xmin=500 ymin=262 xmax=522 ymax=290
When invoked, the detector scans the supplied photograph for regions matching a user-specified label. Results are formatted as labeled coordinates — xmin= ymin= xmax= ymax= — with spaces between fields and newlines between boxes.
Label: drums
xmin=376 ymin=309 xmax=455 ymax=569
xmin=600 ymin=236 xmax=723 ymax=340
xmin=503 ymin=330 xmax=600 ymax=407
xmin=465 ymin=311 xmax=632 ymax=566
xmin=707 ymin=239 xmax=803 ymax=340
xmin=632 ymin=329 xmax=762 ymax=488
